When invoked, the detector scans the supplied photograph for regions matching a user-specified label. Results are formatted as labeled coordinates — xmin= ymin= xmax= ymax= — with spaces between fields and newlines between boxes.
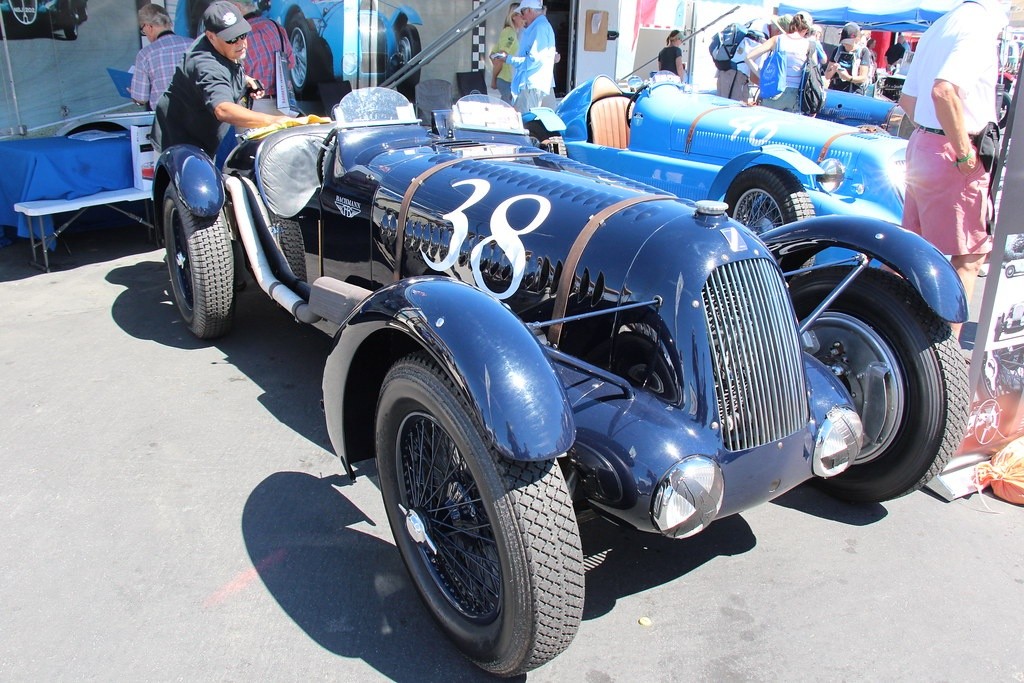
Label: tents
xmin=776 ymin=0 xmax=950 ymax=34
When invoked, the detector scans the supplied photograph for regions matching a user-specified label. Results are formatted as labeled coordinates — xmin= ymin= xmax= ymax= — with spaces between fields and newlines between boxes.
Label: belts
xmin=917 ymin=126 xmax=947 ymax=136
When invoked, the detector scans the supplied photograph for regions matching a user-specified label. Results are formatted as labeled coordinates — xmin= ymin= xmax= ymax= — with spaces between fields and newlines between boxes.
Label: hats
xmin=668 ymin=30 xmax=686 ymax=41
xmin=204 ymin=0 xmax=252 ymax=42
xmin=837 ymin=22 xmax=861 ymax=43
xmin=772 ymin=13 xmax=794 ymax=35
xmin=513 ymin=0 xmax=543 ymax=13
xmin=797 ymin=11 xmax=814 ymax=31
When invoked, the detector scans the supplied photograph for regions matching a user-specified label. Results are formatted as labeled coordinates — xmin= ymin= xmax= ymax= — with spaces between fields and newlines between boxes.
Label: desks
xmin=0 ymin=130 xmax=134 ymax=256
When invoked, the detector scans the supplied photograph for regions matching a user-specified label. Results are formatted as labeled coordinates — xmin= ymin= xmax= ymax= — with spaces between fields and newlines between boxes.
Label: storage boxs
xmin=130 ymin=124 xmax=154 ymax=191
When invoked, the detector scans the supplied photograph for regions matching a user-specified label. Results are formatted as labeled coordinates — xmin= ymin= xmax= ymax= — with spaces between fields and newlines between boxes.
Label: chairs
xmin=588 ymin=95 xmax=636 ymax=150
xmin=318 ymin=80 xmax=353 ymax=117
xmin=414 ymin=79 xmax=453 ymax=126
xmin=456 ymin=70 xmax=489 ymax=103
xmin=257 ymin=120 xmax=343 ymax=219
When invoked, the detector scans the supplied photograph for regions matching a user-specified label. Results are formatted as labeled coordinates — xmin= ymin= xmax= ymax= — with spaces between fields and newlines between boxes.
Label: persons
xmin=885 ymin=36 xmax=905 ymax=67
xmin=499 ymin=0 xmax=557 ymax=112
xmin=879 ymin=0 xmax=1009 ymax=340
xmin=824 ymin=22 xmax=870 ymax=95
xmin=150 ymin=1 xmax=290 ymax=269
xmin=658 ymin=30 xmax=684 ymax=83
xmin=867 ymin=39 xmax=876 ymax=63
xmin=127 ymin=3 xmax=194 ymax=111
xmin=716 ymin=11 xmax=827 ymax=114
xmin=489 ymin=3 xmax=525 ymax=106
xmin=227 ymin=0 xmax=295 ymax=144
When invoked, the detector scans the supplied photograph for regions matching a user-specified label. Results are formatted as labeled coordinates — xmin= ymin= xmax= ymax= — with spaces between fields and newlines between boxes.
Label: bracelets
xmin=849 ymin=78 xmax=853 ymax=82
xmin=957 ymin=151 xmax=972 ymax=162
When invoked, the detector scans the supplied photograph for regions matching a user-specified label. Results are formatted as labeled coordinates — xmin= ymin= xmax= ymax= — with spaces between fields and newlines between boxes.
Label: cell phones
xmin=838 ymin=68 xmax=845 ymax=71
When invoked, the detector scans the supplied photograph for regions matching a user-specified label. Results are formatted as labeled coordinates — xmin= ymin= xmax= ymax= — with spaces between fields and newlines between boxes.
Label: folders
xmin=106 ymin=64 xmax=135 ymax=98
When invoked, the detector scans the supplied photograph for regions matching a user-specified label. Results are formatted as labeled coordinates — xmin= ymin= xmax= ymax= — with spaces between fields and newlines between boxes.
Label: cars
xmin=148 ymin=34 xmax=1015 ymax=680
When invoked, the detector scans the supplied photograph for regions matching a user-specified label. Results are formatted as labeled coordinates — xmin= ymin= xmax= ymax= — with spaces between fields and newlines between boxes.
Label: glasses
xmin=225 ymin=33 xmax=248 ymax=44
xmin=521 ymin=8 xmax=528 ymax=16
xmin=140 ymin=24 xmax=147 ymax=35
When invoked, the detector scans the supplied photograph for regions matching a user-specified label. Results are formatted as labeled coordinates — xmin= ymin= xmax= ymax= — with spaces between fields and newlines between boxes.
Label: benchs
xmin=14 ymin=187 xmax=154 ymax=276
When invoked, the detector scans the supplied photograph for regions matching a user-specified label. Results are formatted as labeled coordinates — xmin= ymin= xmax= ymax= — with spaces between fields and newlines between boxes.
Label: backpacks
xmin=708 ymin=18 xmax=768 ymax=72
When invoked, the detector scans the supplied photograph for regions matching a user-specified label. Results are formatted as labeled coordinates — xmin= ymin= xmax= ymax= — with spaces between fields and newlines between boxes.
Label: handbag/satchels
xmin=799 ymin=59 xmax=824 ymax=119
xmin=757 ymin=34 xmax=787 ymax=102
xmin=273 ymin=49 xmax=300 ymax=118
xmin=977 ymin=121 xmax=1001 ymax=173
